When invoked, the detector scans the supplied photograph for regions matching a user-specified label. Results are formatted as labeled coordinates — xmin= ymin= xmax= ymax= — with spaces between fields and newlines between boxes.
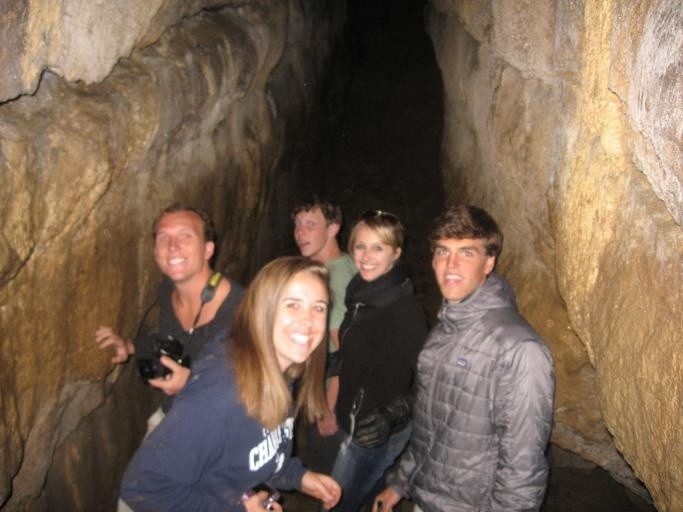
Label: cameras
xmin=139 ymin=335 xmax=189 ymax=381
xmin=243 ymin=483 xmax=281 ymax=511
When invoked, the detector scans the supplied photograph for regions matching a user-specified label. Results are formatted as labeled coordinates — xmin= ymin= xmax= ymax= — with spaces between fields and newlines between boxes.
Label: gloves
xmin=352 ymin=393 xmax=415 ymax=451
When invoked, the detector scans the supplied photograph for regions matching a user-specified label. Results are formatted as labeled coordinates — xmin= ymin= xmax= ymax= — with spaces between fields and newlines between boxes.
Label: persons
xmin=288 ymin=192 xmax=360 ymax=512
xmin=325 ymin=211 xmax=428 ymax=512
xmin=117 ymin=253 xmax=345 ymax=511
xmin=371 ymin=204 xmax=555 ymax=511
xmin=93 ymin=202 xmax=245 ymax=445
